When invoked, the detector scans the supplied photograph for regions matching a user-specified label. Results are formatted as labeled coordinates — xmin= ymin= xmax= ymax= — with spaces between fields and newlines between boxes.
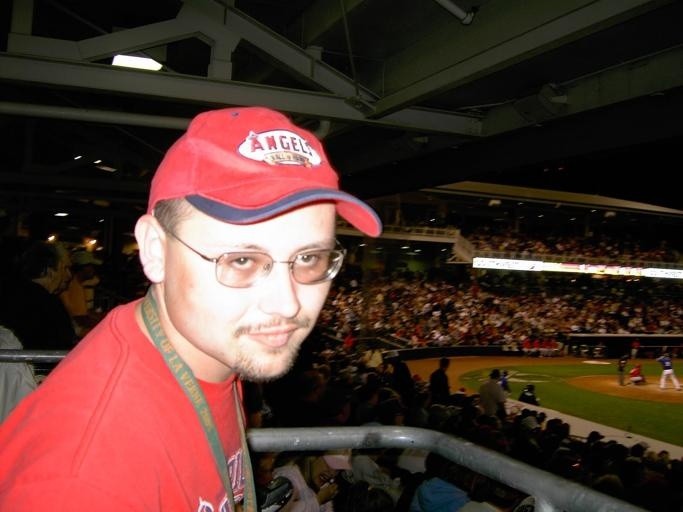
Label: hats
xmin=320 ymin=446 xmax=353 ymax=473
xmin=144 ymin=102 xmax=385 ymax=240
xmin=70 ymin=250 xmax=104 ymax=266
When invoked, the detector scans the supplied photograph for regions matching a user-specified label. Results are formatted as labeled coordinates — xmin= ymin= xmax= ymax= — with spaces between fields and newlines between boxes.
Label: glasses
xmin=156 ymin=219 xmax=349 ymax=290
